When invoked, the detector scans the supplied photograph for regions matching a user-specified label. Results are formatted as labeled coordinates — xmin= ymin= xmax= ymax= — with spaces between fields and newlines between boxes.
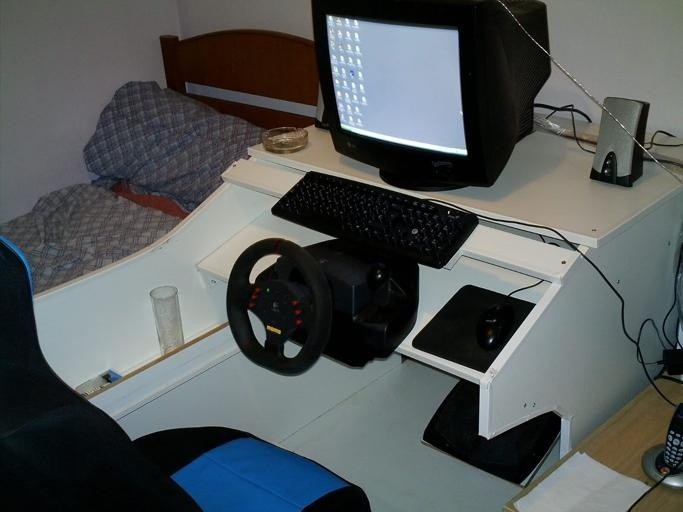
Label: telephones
xmin=656 ymin=404 xmax=683 ymax=475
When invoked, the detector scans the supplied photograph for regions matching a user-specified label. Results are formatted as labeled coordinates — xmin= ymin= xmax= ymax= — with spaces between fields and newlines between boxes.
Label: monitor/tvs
xmin=310 ymin=0 xmax=551 ymax=192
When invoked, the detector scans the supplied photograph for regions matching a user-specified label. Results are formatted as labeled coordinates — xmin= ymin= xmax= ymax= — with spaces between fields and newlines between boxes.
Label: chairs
xmin=1 ymin=236 xmax=371 ymax=512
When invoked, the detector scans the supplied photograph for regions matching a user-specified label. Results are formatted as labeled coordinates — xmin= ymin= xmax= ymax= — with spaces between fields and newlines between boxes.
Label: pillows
xmin=83 ymin=81 xmax=266 ymax=213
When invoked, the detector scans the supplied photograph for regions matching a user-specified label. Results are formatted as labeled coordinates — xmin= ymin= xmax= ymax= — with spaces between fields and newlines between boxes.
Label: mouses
xmin=476 ymin=303 xmax=514 ymax=350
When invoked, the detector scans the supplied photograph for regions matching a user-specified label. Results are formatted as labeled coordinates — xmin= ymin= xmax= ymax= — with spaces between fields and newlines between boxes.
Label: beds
xmin=1 ymin=28 xmax=320 ymax=401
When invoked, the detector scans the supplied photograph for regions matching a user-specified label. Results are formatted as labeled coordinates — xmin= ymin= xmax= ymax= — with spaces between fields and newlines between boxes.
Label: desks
xmin=504 ymin=372 xmax=680 ymax=512
xmin=195 ymin=121 xmax=682 ymax=460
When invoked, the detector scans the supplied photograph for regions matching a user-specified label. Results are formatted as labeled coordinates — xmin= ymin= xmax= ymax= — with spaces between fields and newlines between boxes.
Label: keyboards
xmin=270 ymin=171 xmax=477 ymax=269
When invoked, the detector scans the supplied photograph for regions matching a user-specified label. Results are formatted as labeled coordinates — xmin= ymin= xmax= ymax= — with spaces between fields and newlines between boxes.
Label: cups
xmin=150 ymin=286 xmax=185 ymax=357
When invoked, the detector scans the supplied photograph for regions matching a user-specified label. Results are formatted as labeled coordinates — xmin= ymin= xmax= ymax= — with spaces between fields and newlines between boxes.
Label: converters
xmin=656 ymin=350 xmax=683 ymax=375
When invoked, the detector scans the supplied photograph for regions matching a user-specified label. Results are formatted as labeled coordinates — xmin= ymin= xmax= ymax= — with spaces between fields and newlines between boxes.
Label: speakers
xmin=590 ymin=97 xmax=650 ymax=187
